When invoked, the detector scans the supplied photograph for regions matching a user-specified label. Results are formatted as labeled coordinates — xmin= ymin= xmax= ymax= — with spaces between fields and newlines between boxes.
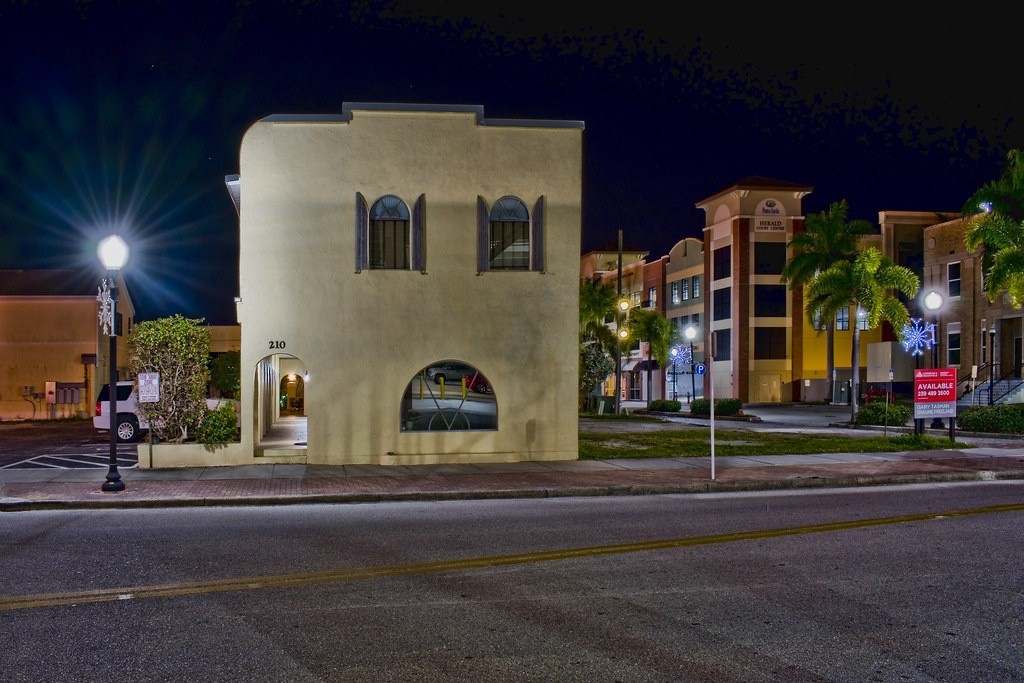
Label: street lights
xmin=616 ymin=298 xmax=629 ymax=416
xmin=897 ymin=316 xmax=936 ymax=436
xmin=671 ymin=348 xmax=678 ymax=400
xmin=684 ymin=326 xmax=696 ymax=399
xmin=924 ymin=290 xmax=946 ymax=429
xmin=96 ymin=233 xmax=129 ymax=492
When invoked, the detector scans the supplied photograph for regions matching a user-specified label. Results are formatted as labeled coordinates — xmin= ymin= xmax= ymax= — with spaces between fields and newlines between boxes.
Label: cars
xmin=427 ymin=363 xmax=475 ymax=385
xmin=462 ymin=373 xmax=491 ymax=394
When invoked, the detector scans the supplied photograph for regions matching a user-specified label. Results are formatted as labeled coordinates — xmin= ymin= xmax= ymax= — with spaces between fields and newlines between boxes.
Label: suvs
xmin=92 ymin=377 xmax=241 ymax=444
